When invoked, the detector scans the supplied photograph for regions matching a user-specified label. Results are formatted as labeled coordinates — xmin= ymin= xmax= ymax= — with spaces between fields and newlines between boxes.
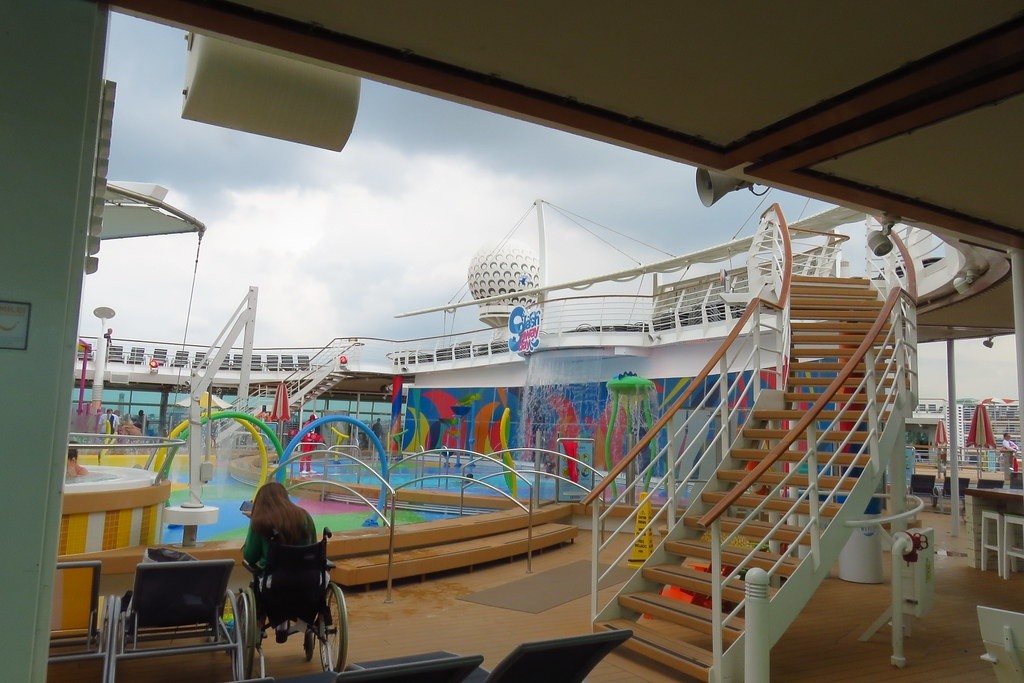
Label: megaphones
xmin=182 ymin=381 xmax=190 ymax=388
xmin=648 ymin=334 xmax=660 ymax=342
xmin=695 ymin=168 xmax=754 ymax=208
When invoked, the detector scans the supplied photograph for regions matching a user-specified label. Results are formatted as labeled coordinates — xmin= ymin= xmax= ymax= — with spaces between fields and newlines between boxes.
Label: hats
xmin=308 ymin=414 xmax=318 ymax=421
xmin=139 ymin=410 xmax=144 ymax=415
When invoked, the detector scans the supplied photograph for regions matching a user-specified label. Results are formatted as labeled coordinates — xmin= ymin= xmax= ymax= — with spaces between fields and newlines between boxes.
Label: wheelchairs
xmin=233 ymin=523 xmax=349 ymax=679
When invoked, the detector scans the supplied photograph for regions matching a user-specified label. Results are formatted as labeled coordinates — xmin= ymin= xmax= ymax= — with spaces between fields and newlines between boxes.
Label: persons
xmin=300 ymin=414 xmax=323 ymax=474
xmin=372 ymin=418 xmax=382 ymax=438
xmin=241 ymin=482 xmax=317 ymax=644
xmin=999 ymin=433 xmax=1018 ymax=471
xmin=97 ymin=409 xmax=149 ymax=438
xmin=460 ymin=473 xmax=473 ymax=484
xmin=104 ymin=328 xmax=112 ymax=346
xmin=545 ymin=454 xmax=556 ymax=478
xmin=67 ymin=441 xmax=88 ymax=476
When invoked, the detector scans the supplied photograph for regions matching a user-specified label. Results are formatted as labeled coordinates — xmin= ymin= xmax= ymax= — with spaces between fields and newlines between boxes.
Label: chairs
xmin=346 ymin=629 xmax=634 ymax=683
xmin=108 ymin=301 xmax=740 ymax=373
xmin=259 ymin=652 xmax=486 ymax=683
xmin=109 ymin=559 xmax=244 ymax=683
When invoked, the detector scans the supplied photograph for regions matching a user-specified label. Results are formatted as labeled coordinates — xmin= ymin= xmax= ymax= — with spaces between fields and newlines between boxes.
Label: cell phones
xmin=239 ymin=501 xmax=254 ymax=511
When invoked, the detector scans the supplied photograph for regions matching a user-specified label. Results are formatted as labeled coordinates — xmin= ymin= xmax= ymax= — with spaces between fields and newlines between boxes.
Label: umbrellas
xmin=935 ymin=419 xmax=948 ymax=478
xmin=270 ymin=380 xmax=290 ymax=441
xmin=175 ymin=391 xmax=232 ymax=418
xmin=966 ymin=404 xmax=996 ymax=479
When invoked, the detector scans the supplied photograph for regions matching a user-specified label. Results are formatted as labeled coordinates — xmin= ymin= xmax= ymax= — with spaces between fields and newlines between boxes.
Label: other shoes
xmin=276 ymin=617 xmax=290 ymax=643
xmin=301 ymin=470 xmax=308 ymax=474
xmin=307 ymin=470 xmax=316 ymax=474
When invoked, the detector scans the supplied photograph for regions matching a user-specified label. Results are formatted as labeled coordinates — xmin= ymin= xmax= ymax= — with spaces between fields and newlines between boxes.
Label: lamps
xmin=868 ymin=212 xmax=906 ymax=255
xmin=983 ymin=333 xmax=996 ymax=347
xmin=952 ymin=266 xmax=976 ymax=294
xmin=180 ymin=32 xmax=361 ymax=152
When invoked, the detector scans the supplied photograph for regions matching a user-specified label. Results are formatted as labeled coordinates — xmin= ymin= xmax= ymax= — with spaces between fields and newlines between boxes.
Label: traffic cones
xmin=627 ymin=492 xmax=654 ymax=570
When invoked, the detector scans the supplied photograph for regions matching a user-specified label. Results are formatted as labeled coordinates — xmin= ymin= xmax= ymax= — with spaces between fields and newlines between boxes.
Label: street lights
xmin=90 ymin=307 xmax=115 ymax=416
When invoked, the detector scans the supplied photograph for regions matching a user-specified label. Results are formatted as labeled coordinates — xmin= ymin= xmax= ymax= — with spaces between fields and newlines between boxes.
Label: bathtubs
xmin=64 ymin=464 xmax=162 ymax=493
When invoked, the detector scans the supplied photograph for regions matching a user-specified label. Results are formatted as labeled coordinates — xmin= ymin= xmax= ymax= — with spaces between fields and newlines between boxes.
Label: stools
xmin=981 ymin=508 xmax=1015 ymax=576
xmin=1001 ymin=512 xmax=1024 ymax=579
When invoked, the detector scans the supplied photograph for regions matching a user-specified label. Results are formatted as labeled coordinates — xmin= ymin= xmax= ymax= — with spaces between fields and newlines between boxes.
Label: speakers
xmin=200 ymin=462 xmax=213 ymax=481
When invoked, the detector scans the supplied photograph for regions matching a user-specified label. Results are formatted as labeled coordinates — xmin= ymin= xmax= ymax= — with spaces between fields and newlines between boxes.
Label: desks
xmin=962 ymin=488 xmax=1024 ymax=570
